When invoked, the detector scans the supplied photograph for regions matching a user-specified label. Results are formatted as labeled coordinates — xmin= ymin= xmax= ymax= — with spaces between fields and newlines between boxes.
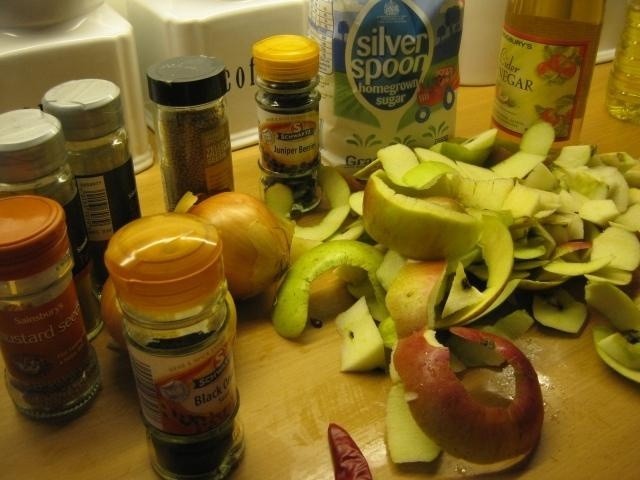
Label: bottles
xmin=252 ymin=34 xmax=322 ymax=217
xmin=40 ymin=79 xmax=144 ymax=281
xmin=147 ymin=55 xmax=235 ymax=211
xmin=1 ymin=196 xmax=101 ymax=421
xmin=489 ymin=0 xmax=606 ymax=165
xmin=0 ymin=109 xmax=103 ymax=341
xmin=106 ymin=214 xmax=244 ymax=480
xmin=606 ymin=0 xmax=640 ymax=122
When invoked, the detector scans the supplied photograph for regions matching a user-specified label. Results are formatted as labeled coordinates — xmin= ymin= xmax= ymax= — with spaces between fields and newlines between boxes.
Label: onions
xmin=99 ymin=273 xmax=128 ymax=351
xmin=186 ymin=192 xmax=292 ymax=301
